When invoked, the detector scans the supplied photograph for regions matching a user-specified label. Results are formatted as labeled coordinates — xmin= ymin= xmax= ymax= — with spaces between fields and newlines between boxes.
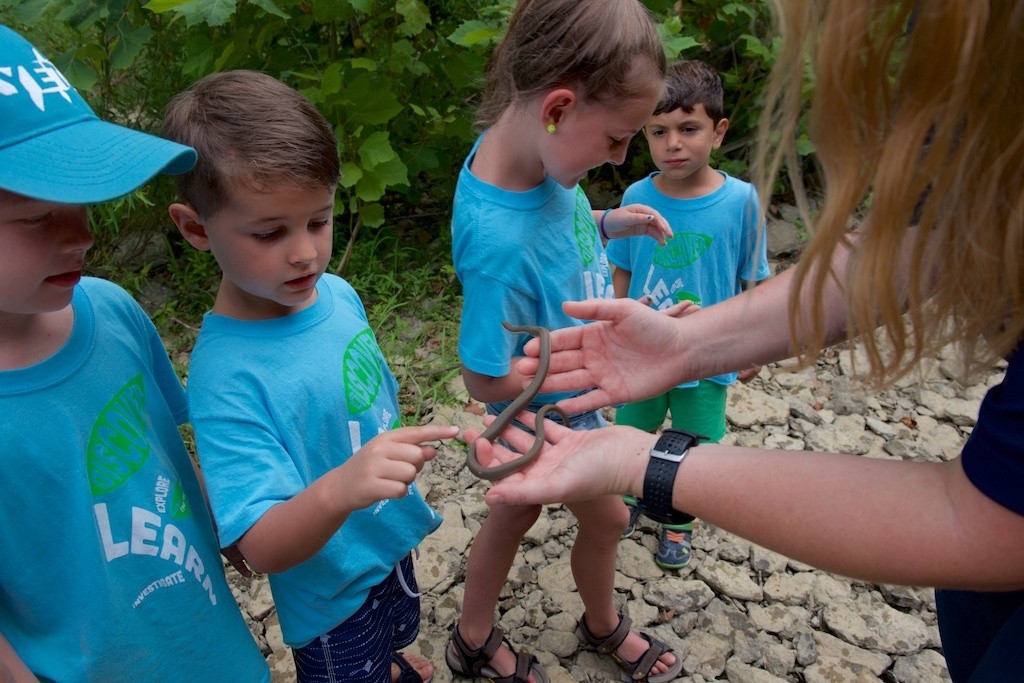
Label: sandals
xmin=390 ymin=652 xmax=434 ymax=683
xmin=444 ymin=621 xmax=551 ymax=683
xmin=579 ymin=610 xmax=683 ymax=683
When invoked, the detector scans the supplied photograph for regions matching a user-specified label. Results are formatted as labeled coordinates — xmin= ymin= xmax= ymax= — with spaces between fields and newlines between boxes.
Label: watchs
xmin=639 ymin=430 xmax=704 ymax=525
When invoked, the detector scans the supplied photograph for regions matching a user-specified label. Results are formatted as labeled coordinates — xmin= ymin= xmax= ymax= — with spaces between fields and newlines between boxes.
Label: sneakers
xmin=619 ymin=504 xmax=640 ymax=539
xmin=654 ymin=522 xmax=693 ymax=570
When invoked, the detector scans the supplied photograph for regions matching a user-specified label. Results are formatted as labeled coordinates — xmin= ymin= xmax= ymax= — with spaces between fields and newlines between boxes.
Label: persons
xmin=445 ymin=0 xmax=687 ymax=683
xmin=163 ymin=72 xmax=460 ymax=681
xmin=606 ymin=63 xmax=768 ymax=567
xmin=0 ymin=23 xmax=272 ymax=681
xmin=462 ymin=0 xmax=1023 ymax=683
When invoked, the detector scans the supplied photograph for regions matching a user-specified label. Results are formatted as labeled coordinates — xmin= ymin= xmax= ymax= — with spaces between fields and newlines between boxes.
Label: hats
xmin=0 ymin=22 xmax=199 ymax=206
